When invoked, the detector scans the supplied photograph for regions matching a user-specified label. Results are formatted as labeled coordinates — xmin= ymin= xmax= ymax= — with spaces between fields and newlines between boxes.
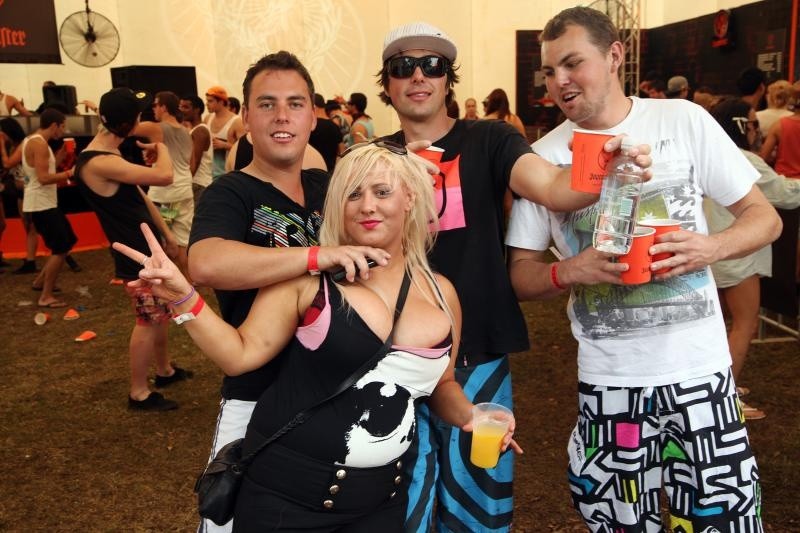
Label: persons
xmin=0 ymin=7 xmax=800 ymax=533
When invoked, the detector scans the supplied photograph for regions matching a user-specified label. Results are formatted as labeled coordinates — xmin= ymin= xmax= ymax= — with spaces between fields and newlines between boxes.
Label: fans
xmin=59 ymin=0 xmax=120 ymax=67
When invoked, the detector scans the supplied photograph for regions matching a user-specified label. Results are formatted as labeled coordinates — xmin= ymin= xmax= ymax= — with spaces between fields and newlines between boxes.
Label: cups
xmin=571 ymin=129 xmax=613 ymax=195
xmin=75 ymin=330 xmax=97 ymax=342
xmin=63 ymin=308 xmax=80 ymax=320
xmin=469 ymin=402 xmax=514 ymax=467
xmin=637 ymin=219 xmax=683 ymax=277
xmin=616 ymin=226 xmax=654 ymax=285
xmin=34 ymin=312 xmax=51 ymax=325
xmin=63 ymin=137 xmax=75 ymax=152
xmin=414 ymin=144 xmax=445 ymax=176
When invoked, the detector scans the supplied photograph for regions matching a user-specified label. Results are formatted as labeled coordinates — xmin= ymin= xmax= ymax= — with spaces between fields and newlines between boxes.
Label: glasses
xmin=339 ymin=140 xmax=407 ymax=157
xmin=388 ymin=56 xmax=449 ymax=79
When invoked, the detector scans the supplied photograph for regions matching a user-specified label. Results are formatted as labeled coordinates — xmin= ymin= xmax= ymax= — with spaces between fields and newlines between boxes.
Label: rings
xmin=141 ymin=256 xmax=147 ymax=265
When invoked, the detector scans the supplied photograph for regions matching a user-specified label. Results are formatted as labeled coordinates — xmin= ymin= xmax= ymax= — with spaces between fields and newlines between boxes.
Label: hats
xmin=382 ymin=24 xmax=457 ymax=64
xmin=206 ymin=88 xmax=228 ymax=107
xmin=98 ymin=89 xmax=153 ymax=126
xmin=668 ymin=75 xmax=690 ymax=93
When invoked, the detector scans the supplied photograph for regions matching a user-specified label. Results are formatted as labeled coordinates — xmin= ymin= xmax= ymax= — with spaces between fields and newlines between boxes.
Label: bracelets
xmin=169 ymin=287 xmax=194 ymax=304
xmin=154 ymin=142 xmax=159 ymax=150
xmin=307 ymin=246 xmax=320 ymax=276
xmin=66 ymin=170 xmax=72 ymax=177
xmin=549 ymin=246 xmax=566 ymax=289
xmin=94 ymin=107 xmax=97 ymax=112
xmin=172 ymin=295 xmax=204 ymax=324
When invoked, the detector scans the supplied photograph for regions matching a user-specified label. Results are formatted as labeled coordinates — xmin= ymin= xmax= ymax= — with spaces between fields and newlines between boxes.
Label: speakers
xmin=43 ymin=85 xmax=77 ymax=114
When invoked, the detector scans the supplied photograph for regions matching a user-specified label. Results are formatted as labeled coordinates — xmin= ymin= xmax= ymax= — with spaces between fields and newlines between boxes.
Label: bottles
xmin=591 ymin=136 xmax=647 ymax=254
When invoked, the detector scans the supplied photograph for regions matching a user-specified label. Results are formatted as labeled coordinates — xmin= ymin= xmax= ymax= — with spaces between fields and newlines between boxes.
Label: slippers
xmin=736 ymin=386 xmax=750 ymax=397
xmin=32 ymin=282 xmax=59 ymax=293
xmin=37 ymin=299 xmax=68 ymax=308
xmin=740 ymin=401 xmax=766 ymax=420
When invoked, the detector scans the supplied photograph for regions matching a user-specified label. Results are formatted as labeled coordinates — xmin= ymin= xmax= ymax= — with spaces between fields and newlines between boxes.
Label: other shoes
xmin=66 ymin=256 xmax=81 ymax=273
xmin=127 ymin=389 xmax=178 ymax=411
xmin=155 ymin=363 xmax=192 ymax=387
xmin=15 ymin=261 xmax=38 ymax=275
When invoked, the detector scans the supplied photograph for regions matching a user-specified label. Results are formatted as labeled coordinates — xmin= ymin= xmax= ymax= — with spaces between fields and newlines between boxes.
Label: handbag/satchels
xmin=192 ymin=437 xmax=250 ymax=526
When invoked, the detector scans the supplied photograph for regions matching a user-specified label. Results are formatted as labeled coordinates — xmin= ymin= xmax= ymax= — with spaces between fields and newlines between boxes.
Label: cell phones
xmin=332 ymin=259 xmax=377 ymax=282
